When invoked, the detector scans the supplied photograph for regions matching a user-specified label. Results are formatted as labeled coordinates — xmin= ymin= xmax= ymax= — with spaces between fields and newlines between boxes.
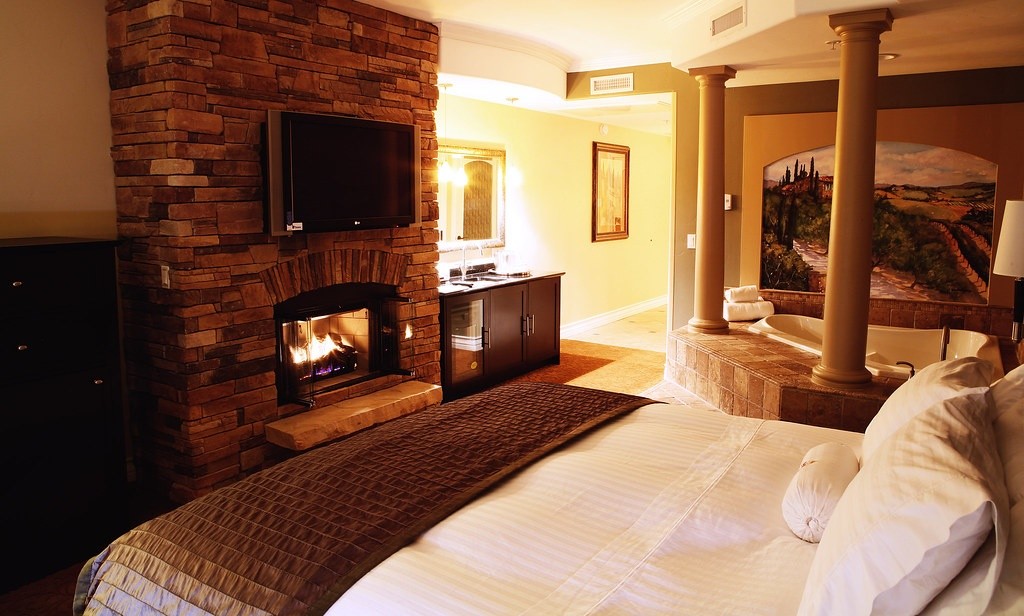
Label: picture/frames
xmin=592 ymin=141 xmax=631 ymax=242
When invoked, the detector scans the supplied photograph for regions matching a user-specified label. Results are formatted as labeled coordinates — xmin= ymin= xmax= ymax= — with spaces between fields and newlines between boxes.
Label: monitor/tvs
xmin=280 ymin=110 xmax=415 ymax=235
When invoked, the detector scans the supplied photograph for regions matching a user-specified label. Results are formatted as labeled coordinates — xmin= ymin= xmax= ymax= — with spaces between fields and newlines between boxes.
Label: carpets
xmin=477 ymin=340 xmax=667 ymax=397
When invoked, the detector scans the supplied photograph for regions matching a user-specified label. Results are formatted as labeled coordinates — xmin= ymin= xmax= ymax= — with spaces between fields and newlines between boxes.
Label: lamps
xmin=992 ymin=200 xmax=1024 ymax=341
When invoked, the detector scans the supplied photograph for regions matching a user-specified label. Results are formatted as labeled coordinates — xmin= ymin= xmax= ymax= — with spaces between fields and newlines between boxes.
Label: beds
xmin=73 ymin=380 xmax=864 ymax=616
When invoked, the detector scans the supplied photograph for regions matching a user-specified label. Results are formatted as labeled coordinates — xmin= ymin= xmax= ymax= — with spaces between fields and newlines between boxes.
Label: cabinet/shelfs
xmin=1 ymin=238 xmax=129 ymax=596
xmin=441 ymin=269 xmax=565 ymax=399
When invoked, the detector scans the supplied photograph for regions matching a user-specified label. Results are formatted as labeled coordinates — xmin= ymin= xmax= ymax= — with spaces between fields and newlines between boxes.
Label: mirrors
xmin=437 ymin=146 xmax=506 ymax=254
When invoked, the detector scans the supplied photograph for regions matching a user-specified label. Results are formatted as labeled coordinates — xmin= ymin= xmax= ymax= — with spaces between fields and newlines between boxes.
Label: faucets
xmin=461 ymin=242 xmax=484 ymax=276
xmin=939 ymin=324 xmax=951 ymax=363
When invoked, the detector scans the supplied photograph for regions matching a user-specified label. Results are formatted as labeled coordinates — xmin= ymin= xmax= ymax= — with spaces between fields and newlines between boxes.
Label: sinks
xmin=462 ymin=278 xmax=506 ymax=284
xmin=436 ymin=282 xmax=472 ymax=294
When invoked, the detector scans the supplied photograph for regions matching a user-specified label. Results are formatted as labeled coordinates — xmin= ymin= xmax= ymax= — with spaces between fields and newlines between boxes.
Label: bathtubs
xmin=747 ymin=313 xmax=992 ymax=380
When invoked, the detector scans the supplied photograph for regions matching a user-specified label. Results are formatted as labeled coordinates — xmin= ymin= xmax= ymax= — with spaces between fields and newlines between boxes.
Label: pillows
xmin=781 ymin=441 xmax=860 ymax=543
xmin=860 ymin=357 xmax=1001 ymax=467
xmin=978 ymin=362 xmax=1024 ymax=616
xmin=796 ymin=386 xmax=1010 ymax=616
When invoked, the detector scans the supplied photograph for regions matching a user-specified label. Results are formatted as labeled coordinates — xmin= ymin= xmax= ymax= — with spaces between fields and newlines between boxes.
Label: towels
xmin=722 ymin=285 xmax=775 ymax=322
